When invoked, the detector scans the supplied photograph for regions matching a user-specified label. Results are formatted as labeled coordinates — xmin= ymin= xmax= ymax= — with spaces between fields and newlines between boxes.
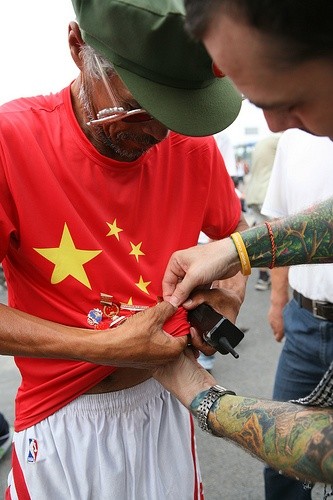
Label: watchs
xmin=196 ymin=384 xmax=236 ymax=438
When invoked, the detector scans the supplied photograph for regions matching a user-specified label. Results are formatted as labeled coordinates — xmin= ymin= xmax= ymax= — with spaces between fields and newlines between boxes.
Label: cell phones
xmin=186 ymin=303 xmax=245 ymax=359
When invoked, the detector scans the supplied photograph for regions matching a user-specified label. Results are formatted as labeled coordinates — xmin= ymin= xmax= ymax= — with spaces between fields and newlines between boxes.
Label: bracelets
xmin=231 ymin=231 xmax=252 ymax=277
xmin=263 ymin=220 xmax=277 ymax=270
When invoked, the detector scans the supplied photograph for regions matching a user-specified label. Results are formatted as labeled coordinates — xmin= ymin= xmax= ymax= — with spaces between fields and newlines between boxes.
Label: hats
xmin=72 ymin=0 xmax=242 ymax=136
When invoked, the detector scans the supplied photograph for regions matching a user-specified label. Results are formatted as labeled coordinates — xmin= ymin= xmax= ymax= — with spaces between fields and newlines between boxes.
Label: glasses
xmin=88 ymin=45 xmax=156 ymax=126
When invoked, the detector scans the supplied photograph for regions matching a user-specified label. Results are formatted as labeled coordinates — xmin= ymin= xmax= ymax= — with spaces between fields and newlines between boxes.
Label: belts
xmin=293 ymin=288 xmax=333 ymax=321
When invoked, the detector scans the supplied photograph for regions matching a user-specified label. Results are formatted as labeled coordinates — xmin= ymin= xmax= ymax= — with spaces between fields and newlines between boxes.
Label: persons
xmin=0 ymin=0 xmax=241 ymax=500
xmin=259 ymin=126 xmax=332 ymax=500
xmin=151 ymin=0 xmax=332 ymax=485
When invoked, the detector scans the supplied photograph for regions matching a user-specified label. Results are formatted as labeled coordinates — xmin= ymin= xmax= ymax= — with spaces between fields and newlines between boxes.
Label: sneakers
xmin=255 ymin=275 xmax=271 ymax=291
